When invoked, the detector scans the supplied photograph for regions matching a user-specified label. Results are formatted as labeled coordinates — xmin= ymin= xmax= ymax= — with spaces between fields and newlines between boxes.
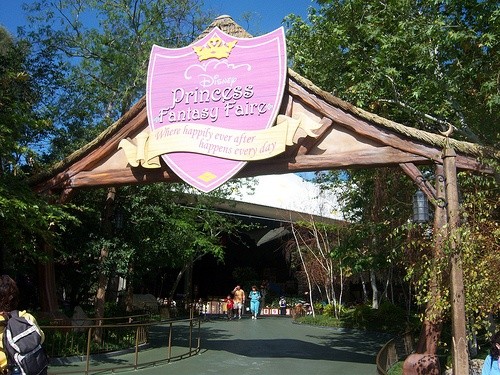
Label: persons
xmin=481 ymin=330 xmax=500 ymax=375
xmin=232 ymin=286 xmax=245 ymax=319
xmin=225 ymin=294 xmax=235 ymax=320
xmin=279 ymin=296 xmax=286 ymax=315
xmin=249 ymin=285 xmax=261 ymax=319
xmin=0 ymin=274 xmax=46 ymax=375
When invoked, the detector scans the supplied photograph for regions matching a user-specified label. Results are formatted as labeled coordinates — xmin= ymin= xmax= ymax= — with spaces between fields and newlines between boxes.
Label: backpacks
xmin=3 ymin=310 xmax=48 ymax=375
